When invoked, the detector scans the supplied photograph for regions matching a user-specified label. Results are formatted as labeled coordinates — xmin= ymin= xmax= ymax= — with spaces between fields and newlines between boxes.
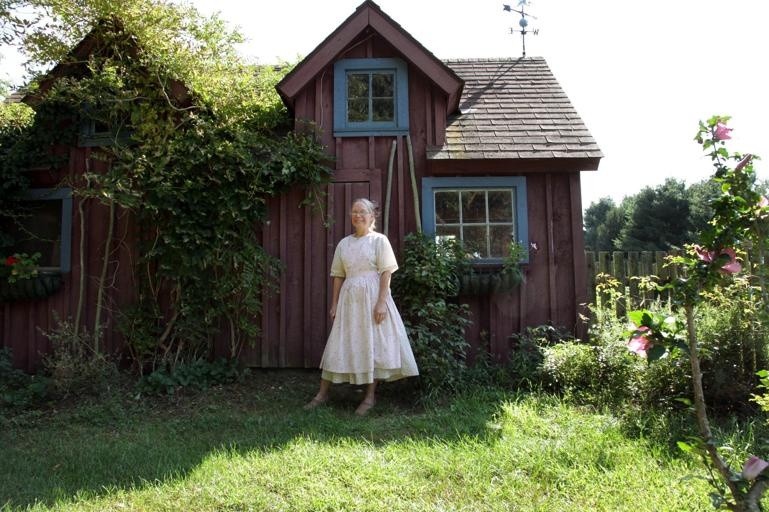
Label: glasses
xmin=351 ymin=209 xmax=370 ymax=217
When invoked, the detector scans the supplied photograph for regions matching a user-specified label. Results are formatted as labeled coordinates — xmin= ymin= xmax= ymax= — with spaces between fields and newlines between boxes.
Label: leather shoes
xmin=302 ymin=394 xmax=329 ymax=411
xmin=354 ymin=396 xmax=377 ymax=416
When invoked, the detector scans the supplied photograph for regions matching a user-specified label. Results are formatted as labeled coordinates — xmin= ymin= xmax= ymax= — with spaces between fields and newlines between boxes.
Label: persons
xmin=303 ymin=198 xmax=420 ymax=417
